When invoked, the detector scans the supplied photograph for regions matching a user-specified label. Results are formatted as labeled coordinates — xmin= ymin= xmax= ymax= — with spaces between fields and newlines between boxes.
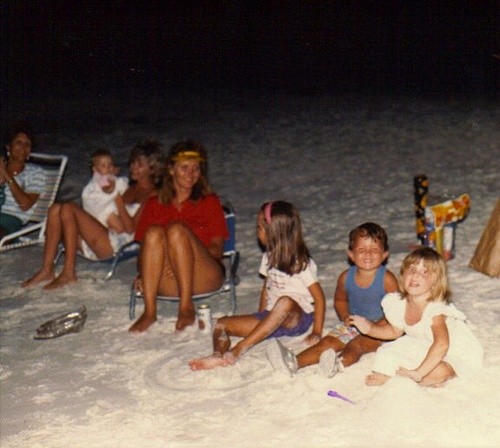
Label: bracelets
xmin=8 ymin=177 xmax=15 ymax=184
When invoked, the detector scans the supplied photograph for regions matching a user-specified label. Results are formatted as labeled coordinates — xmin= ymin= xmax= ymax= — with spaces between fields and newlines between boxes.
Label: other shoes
xmin=318 ymin=349 xmax=344 ymax=378
xmin=33 ymin=306 xmax=87 ymax=340
xmin=267 ymin=337 xmax=298 ymax=378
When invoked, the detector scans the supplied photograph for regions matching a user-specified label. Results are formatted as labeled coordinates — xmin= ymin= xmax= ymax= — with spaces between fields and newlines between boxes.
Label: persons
xmin=0 ymin=131 xmax=43 ymax=244
xmin=22 ymin=144 xmax=162 ymax=290
xmin=267 ymin=222 xmax=399 ymax=379
xmin=128 ymin=141 xmax=226 ymax=333
xmin=189 ymin=200 xmax=326 ymax=372
xmin=81 ymin=149 xmax=129 ymax=235
xmin=350 ymin=245 xmax=483 ymax=387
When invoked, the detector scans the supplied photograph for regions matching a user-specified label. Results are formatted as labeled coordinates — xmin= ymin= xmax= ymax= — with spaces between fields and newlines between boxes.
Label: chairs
xmin=0 ymin=152 xmax=68 ymax=251
xmin=52 ymin=234 xmax=140 ymax=281
xmin=128 ymin=196 xmax=241 ymax=320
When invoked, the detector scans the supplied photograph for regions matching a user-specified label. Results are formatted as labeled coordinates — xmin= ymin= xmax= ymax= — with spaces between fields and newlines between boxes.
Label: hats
xmin=172 ymin=150 xmax=205 ymax=162
